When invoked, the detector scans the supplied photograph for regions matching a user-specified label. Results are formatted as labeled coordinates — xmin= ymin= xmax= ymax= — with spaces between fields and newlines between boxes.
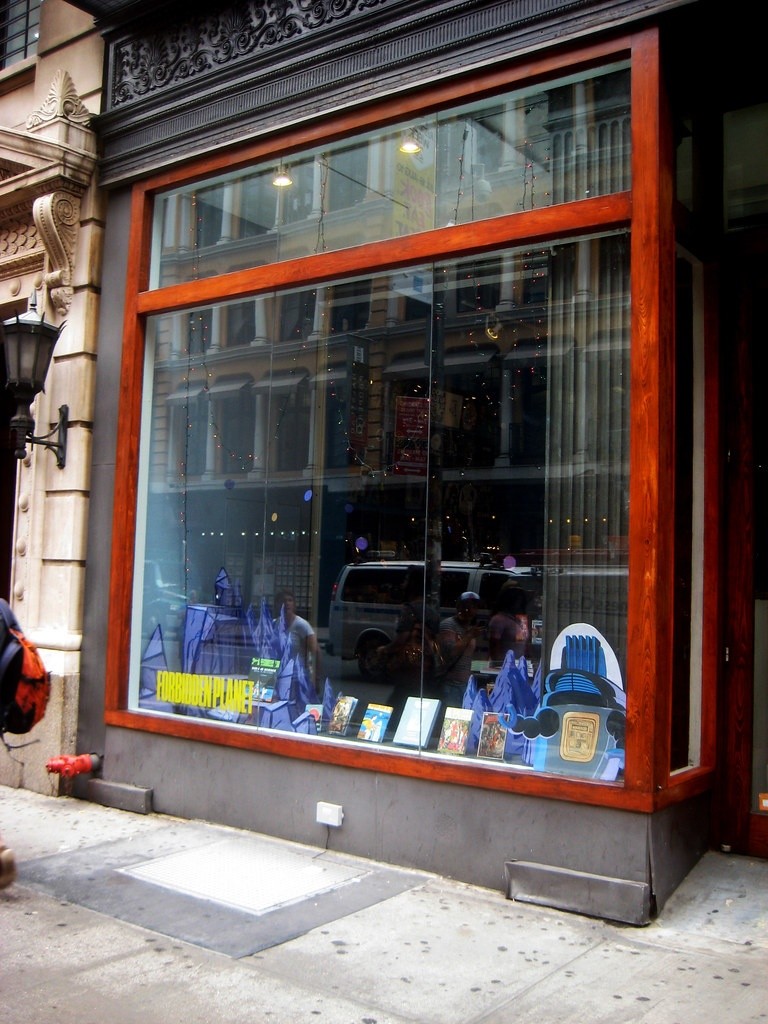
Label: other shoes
xmin=0 ymin=846 xmax=17 ymax=889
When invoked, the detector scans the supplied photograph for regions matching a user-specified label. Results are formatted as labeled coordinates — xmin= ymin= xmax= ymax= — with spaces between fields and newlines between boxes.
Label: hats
xmin=457 ymin=591 xmax=480 ymax=603
xmin=501 ymin=581 xmax=536 ymax=605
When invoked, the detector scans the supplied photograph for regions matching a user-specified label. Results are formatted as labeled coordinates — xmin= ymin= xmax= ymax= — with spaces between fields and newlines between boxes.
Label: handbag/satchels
xmin=429 ymin=662 xmax=455 ymax=689
xmin=387 ymin=625 xmax=447 ymax=678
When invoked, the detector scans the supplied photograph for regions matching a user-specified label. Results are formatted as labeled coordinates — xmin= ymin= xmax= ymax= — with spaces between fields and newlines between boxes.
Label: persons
xmin=0 ymin=597 xmax=23 ymax=889
xmin=271 ymin=591 xmax=323 ymax=703
xmin=388 ymin=587 xmax=528 ymax=731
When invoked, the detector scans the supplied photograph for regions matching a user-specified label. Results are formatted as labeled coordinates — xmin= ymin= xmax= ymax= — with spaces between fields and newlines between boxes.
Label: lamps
xmin=272 ymin=152 xmax=292 ymax=187
xmin=399 ymin=129 xmax=421 ymax=154
xmin=1 ymin=286 xmax=69 ymax=468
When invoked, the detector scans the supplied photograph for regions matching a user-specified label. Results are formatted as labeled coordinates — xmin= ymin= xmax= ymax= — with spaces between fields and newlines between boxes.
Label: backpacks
xmin=0 ymin=608 xmax=51 ymax=766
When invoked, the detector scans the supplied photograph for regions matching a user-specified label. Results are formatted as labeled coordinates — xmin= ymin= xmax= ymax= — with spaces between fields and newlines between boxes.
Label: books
xmin=327 ymin=695 xmax=473 ymax=755
